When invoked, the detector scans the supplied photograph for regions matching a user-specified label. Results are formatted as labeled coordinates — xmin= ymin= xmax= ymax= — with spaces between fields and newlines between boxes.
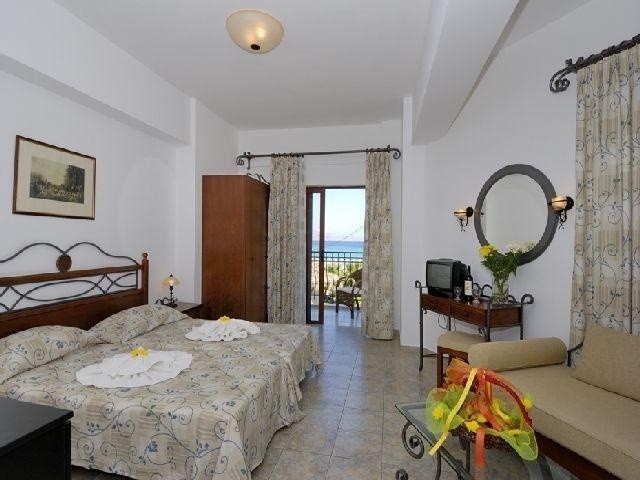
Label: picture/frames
xmin=12 ymin=133 xmax=98 ymax=221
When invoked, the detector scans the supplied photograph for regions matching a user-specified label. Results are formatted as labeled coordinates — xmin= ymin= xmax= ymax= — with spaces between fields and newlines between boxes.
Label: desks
xmin=411 ymin=279 xmax=534 ymax=372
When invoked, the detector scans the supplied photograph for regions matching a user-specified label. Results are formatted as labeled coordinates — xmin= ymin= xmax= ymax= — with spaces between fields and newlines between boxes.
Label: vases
xmin=491 ymin=276 xmax=509 ymax=305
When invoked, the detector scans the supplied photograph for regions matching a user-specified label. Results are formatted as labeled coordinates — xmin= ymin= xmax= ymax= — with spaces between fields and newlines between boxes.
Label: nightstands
xmin=0 ymin=393 xmax=75 ymax=480
xmin=163 ymin=300 xmax=203 ymax=319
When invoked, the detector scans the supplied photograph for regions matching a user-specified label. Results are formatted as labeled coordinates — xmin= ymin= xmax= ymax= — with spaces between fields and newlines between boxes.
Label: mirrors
xmin=473 ymin=162 xmax=559 ymax=269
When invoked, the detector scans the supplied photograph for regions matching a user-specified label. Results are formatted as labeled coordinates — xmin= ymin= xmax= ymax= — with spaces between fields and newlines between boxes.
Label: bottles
xmin=464 ymin=264 xmax=473 ymax=304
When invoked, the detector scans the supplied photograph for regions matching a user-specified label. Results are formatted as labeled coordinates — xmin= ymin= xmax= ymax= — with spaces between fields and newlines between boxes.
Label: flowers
xmin=476 ymin=239 xmax=534 ymax=300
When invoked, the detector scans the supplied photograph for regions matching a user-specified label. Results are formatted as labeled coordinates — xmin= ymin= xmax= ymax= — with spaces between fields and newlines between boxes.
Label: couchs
xmin=486 ymin=322 xmax=640 ymax=480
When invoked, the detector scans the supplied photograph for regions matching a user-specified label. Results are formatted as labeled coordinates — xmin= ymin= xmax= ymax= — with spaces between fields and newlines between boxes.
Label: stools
xmin=435 ymin=330 xmax=487 ymax=388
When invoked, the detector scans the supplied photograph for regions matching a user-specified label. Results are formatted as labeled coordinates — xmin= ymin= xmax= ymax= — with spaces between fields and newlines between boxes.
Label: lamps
xmin=546 ymin=194 xmax=574 ymax=224
xmin=452 ymin=206 xmax=475 ymax=230
xmin=226 ymin=7 xmax=284 ymax=56
xmin=154 ymin=272 xmax=179 ymax=308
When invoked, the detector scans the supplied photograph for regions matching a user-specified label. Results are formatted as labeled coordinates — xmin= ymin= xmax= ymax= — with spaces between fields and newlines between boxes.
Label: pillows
xmin=0 ymin=305 xmax=191 ymax=385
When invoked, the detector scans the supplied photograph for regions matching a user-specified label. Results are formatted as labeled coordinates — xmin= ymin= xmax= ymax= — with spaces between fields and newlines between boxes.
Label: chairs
xmin=335 ymin=268 xmax=363 ymax=319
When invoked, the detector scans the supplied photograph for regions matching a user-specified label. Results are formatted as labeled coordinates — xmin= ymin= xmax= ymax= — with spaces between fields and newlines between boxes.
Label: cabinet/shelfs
xmin=201 ymin=172 xmax=267 ymax=322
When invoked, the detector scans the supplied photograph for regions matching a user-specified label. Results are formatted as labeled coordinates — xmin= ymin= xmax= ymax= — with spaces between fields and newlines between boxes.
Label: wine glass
xmin=472 ymin=289 xmax=481 ymax=305
xmin=452 ymin=286 xmax=462 ymax=301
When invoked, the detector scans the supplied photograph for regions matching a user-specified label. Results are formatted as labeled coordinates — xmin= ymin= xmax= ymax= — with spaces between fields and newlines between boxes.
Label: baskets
xmin=443 ymin=369 xmax=532 ymax=450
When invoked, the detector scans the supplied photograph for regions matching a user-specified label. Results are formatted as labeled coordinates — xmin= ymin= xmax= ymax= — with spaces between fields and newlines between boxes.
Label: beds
xmin=0 ymin=239 xmax=315 ymax=480
